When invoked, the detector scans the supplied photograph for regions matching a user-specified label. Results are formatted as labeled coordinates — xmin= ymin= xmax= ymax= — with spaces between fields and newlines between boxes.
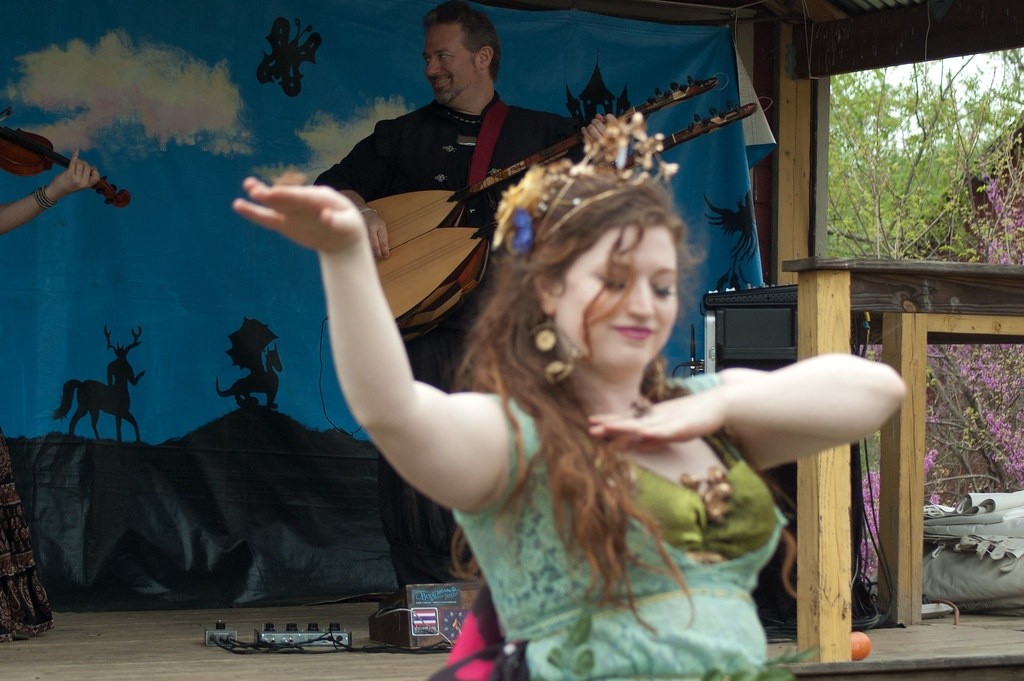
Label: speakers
xmin=700 ymin=306 xmax=865 ymax=626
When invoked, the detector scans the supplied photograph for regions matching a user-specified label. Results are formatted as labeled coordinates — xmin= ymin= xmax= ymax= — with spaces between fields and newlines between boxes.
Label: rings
xmin=631 ymin=398 xmax=652 ymax=419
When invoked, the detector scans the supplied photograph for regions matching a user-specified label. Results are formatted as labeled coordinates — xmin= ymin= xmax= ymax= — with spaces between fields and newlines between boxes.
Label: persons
xmin=231 ymin=112 xmax=907 ymax=681
xmin=312 ymin=0 xmax=622 ymax=608
xmin=0 ymin=148 xmax=100 ymax=234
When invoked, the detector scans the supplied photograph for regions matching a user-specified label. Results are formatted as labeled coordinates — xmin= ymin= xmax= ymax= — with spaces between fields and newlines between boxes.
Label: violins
xmin=1 ymin=109 xmax=130 ymax=208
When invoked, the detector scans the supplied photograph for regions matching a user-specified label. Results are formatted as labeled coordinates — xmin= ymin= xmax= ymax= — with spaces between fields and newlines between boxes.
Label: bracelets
xmin=358 ymin=206 xmax=378 ymax=215
xmin=34 ymin=185 xmax=56 ymax=209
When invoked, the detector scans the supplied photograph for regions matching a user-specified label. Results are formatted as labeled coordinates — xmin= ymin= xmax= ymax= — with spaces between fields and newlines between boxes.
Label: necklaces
xmin=448 ymin=111 xmax=482 ymax=124
